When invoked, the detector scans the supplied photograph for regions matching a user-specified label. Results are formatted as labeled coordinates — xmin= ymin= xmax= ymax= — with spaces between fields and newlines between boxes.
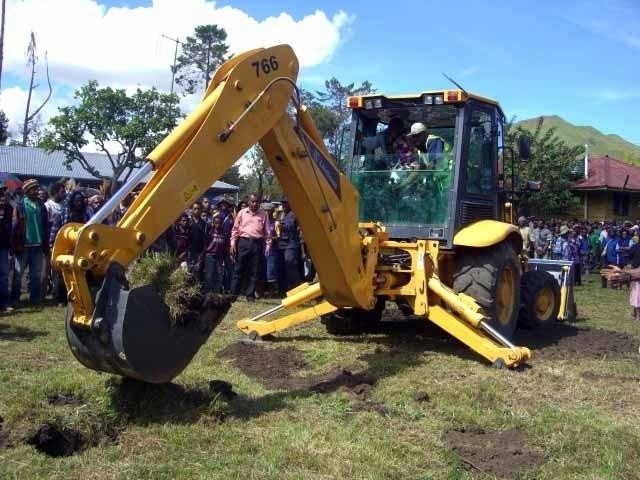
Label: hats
xmin=558 ymin=225 xmax=572 ymax=235
xmin=23 ymin=178 xmax=39 ymax=194
xmin=404 ymin=122 xmax=428 ymax=137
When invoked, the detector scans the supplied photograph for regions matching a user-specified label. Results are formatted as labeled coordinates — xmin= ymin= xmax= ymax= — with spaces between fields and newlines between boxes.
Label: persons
xmin=511 ymin=216 xmax=640 ymax=319
xmin=147 ymin=191 xmax=314 ymax=300
xmin=363 ymin=117 xmax=453 ymax=225
xmin=0 ymin=176 xmax=147 ymax=315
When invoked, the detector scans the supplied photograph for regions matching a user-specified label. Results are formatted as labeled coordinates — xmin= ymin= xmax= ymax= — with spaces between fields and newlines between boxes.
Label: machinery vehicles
xmin=49 ymin=44 xmax=580 ymax=384
